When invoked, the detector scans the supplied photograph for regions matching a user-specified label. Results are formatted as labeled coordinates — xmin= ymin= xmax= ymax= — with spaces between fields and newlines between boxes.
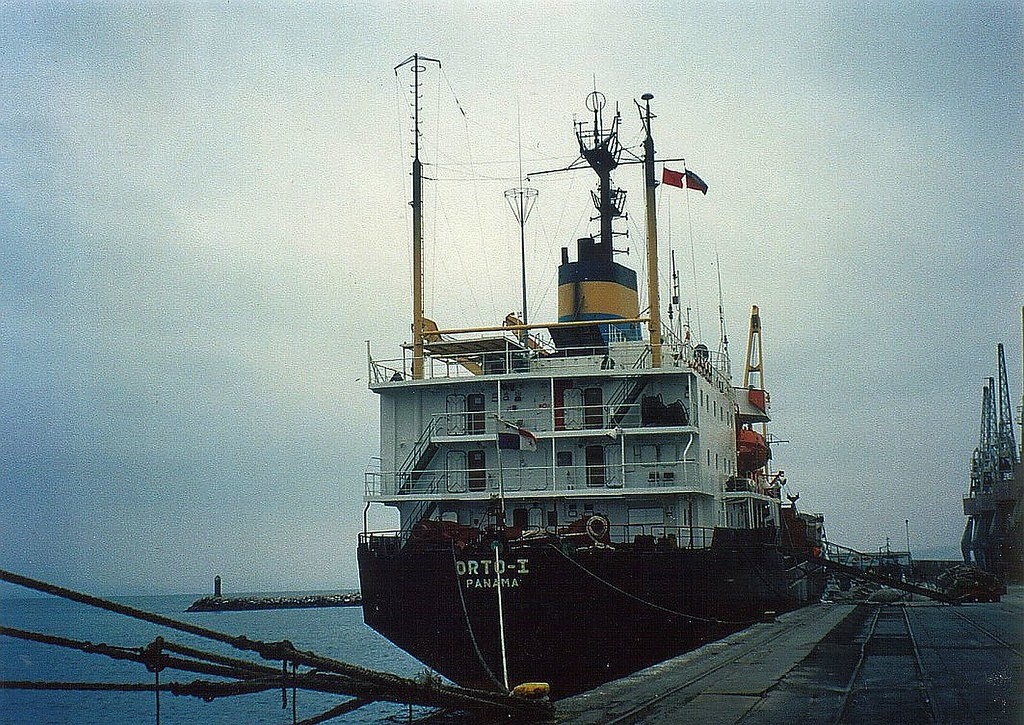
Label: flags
xmin=685 ymin=169 xmax=708 ymax=195
xmin=496 ymin=429 xmax=537 ymax=452
xmin=749 ymin=390 xmax=765 ymax=412
xmin=662 ymin=168 xmax=685 ymax=188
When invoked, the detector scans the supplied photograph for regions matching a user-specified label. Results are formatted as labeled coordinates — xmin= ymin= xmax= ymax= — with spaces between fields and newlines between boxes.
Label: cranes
xmin=955 ymin=335 xmax=1024 ymax=589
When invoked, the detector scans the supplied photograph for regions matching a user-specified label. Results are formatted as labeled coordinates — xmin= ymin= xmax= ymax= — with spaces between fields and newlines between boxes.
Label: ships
xmin=346 ymin=41 xmax=836 ymax=689
